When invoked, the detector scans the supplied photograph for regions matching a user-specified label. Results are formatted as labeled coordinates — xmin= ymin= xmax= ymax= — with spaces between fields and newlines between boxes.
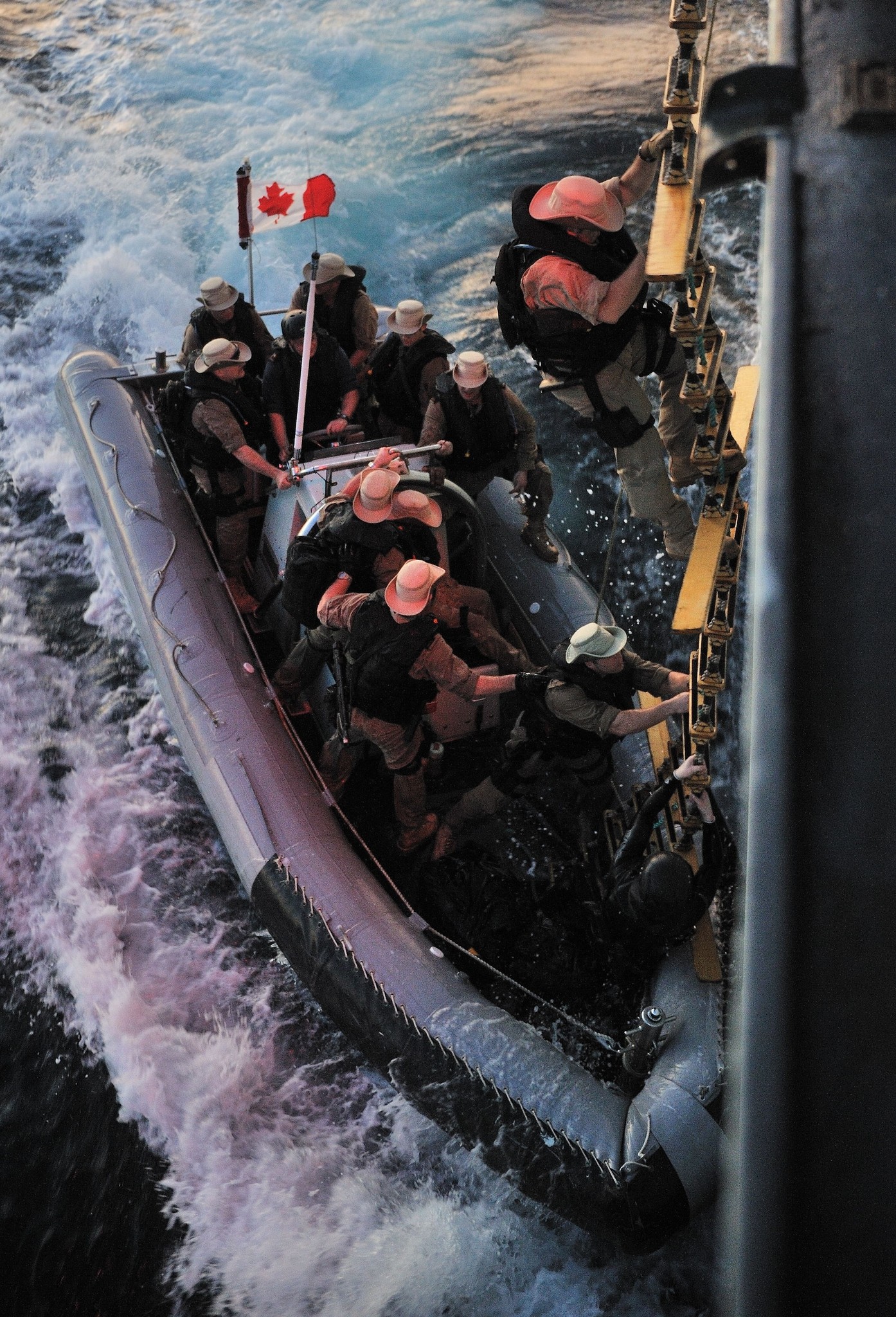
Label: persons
xmin=573 ymin=753 xmax=742 ymax=1002
xmin=183 ymin=254 xmax=692 ymax=864
xmin=512 ymin=129 xmax=747 ymax=559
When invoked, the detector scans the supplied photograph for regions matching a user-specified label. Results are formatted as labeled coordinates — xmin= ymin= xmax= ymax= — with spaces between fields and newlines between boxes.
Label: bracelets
xmin=336 ymin=413 xmax=349 ymax=421
xmin=638 ymin=140 xmax=657 ymax=163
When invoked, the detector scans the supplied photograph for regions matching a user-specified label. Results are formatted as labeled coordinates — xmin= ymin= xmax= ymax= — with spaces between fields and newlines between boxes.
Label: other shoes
xmin=223 ymin=577 xmax=257 ymax=614
xmin=399 ymin=812 xmax=440 ymax=851
xmin=431 ymin=822 xmax=459 ymax=860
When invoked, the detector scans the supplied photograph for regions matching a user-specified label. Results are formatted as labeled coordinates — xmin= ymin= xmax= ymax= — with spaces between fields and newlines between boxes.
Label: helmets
xmin=642 ymin=850 xmax=694 ymax=913
xmin=281 ymin=309 xmax=318 ymax=341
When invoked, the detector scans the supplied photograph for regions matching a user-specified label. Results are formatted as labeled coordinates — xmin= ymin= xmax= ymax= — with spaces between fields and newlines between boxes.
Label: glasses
xmin=230 ymin=343 xmax=240 ymax=360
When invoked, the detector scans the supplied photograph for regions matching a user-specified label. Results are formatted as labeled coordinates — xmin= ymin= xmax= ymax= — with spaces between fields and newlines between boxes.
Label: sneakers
xmin=669 ymin=450 xmax=747 ymax=488
xmin=665 ymin=530 xmax=740 ymax=560
xmin=521 ymin=522 xmax=559 ymax=564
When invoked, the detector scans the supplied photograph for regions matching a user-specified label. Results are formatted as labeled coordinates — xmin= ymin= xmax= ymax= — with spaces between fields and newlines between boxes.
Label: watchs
xmin=338 ymin=571 xmax=352 ymax=582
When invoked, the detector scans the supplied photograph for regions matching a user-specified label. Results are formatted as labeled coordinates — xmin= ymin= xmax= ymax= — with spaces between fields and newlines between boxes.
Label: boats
xmin=59 ymin=300 xmax=733 ymax=1213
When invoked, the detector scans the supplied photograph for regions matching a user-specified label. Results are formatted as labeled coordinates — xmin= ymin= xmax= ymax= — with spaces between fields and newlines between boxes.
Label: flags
xmin=247 ymin=173 xmax=336 ymax=238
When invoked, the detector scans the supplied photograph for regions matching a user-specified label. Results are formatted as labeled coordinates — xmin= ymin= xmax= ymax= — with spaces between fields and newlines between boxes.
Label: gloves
xmin=337 ymin=541 xmax=370 ymax=578
xmin=637 ymin=129 xmax=673 ymax=162
xmin=514 ymin=665 xmax=550 ymax=696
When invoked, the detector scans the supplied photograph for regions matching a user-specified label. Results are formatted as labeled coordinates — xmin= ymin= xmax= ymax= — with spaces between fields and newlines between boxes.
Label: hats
xmin=303 ymin=254 xmax=355 ymax=283
xmin=353 ymin=467 xmax=400 ymax=523
xmin=529 ymin=175 xmax=624 ymax=232
xmin=194 ymin=338 xmax=253 ymax=373
xmin=384 ymin=559 xmax=446 ymax=616
xmin=453 ymin=352 xmax=490 ymax=389
xmin=195 ymin=278 xmax=239 ymax=312
xmin=566 ymin=622 xmax=627 ymax=664
xmin=387 ymin=490 xmax=442 ymax=528
xmin=386 ymin=299 xmax=434 ymax=335
